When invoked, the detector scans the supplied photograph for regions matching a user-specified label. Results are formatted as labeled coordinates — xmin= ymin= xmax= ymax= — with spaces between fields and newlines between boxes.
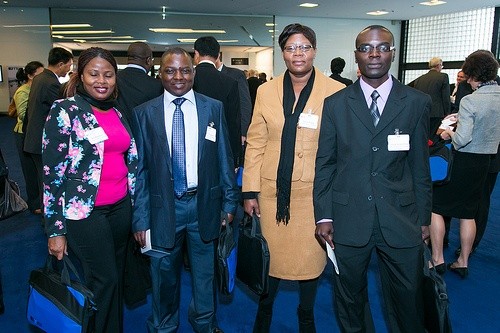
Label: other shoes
xmin=448 ymin=262 xmax=468 ymax=277
xmin=430 ymin=263 xmax=446 ymax=273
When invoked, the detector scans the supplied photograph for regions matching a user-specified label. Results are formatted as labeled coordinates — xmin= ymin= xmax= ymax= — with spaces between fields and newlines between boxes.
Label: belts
xmin=182 ymin=188 xmax=198 ymax=197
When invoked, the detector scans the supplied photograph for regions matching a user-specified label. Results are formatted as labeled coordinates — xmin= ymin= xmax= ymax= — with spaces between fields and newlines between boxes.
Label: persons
xmin=130 ymin=47 xmax=240 ymax=333
xmin=192 ymin=36 xmax=252 ymax=174
xmin=244 ymin=70 xmax=267 ymax=105
xmin=241 ymin=23 xmax=347 ymax=333
xmin=449 ymin=71 xmax=466 ymax=98
xmin=41 ymin=47 xmax=138 ymax=333
xmin=13 ymin=62 xmax=44 ymax=214
xmin=329 ymin=57 xmax=353 ymax=87
xmin=21 ymin=47 xmax=73 ymax=191
xmin=313 ymin=25 xmax=433 ymax=333
xmin=429 ymin=50 xmax=500 ymax=279
xmin=117 ymin=41 xmax=164 ymax=117
xmin=414 ymin=57 xmax=451 ymax=143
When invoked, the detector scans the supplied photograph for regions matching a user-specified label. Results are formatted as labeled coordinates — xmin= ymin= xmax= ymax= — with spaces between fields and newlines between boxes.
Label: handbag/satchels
xmin=7 ymin=100 xmax=17 ymax=117
xmin=219 ymin=214 xmax=237 ymax=304
xmin=238 ymin=212 xmax=271 ymax=297
xmin=26 ymin=254 xmax=95 ymax=333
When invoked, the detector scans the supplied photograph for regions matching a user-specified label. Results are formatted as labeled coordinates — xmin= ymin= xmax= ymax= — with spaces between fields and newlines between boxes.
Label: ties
xmin=371 ymin=91 xmax=380 ymax=128
xmin=172 ymin=99 xmax=187 ymax=197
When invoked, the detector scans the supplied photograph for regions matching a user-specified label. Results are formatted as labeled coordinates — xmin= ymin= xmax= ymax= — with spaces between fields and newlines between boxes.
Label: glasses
xmin=357 ymin=45 xmax=395 ymax=53
xmin=285 ymin=45 xmax=312 ymax=52
